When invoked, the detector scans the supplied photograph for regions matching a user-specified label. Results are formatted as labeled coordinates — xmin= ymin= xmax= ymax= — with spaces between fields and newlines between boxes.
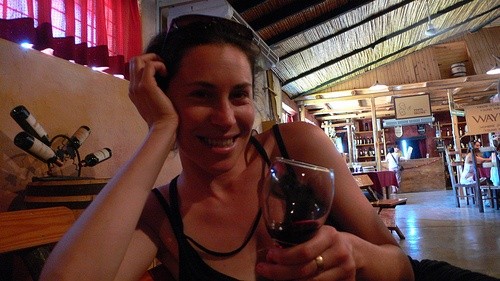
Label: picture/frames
xmin=394 ymin=93 xmax=432 ymax=120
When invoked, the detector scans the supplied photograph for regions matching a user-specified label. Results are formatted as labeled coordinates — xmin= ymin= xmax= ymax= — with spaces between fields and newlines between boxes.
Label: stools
xmin=374 ymin=207 xmax=405 ymax=240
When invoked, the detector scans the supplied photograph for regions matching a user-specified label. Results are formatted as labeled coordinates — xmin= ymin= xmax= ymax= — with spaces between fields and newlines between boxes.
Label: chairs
xmin=445 ymin=150 xmax=487 ymax=208
xmin=472 ymin=148 xmax=500 ymax=213
xmin=1 ymin=205 xmax=77 ymax=281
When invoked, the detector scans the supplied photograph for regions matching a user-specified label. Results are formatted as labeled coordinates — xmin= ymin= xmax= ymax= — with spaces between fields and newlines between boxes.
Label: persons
xmin=386 ymin=147 xmax=402 ymax=193
xmin=459 ymin=139 xmax=491 ymax=186
xmin=474 ymin=148 xmax=486 ymax=179
xmin=394 ymin=147 xmax=402 ymax=158
xmin=490 ymin=136 xmax=500 ymax=190
xmin=39 ymin=14 xmax=497 ymax=280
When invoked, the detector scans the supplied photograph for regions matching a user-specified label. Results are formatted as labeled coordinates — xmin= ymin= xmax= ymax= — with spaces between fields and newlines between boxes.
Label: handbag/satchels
xmin=390 ymin=153 xmax=404 ymax=171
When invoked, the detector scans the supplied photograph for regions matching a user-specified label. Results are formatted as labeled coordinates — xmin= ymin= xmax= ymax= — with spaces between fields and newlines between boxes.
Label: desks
xmin=22 ymin=176 xmax=114 ymax=220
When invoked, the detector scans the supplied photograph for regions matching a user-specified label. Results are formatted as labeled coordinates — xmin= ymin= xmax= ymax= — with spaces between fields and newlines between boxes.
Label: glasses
xmin=163 ymin=13 xmax=254 ymax=78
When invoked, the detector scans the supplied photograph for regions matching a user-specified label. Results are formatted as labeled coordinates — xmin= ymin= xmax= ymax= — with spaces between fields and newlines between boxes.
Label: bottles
xmin=10 ymin=105 xmax=52 ymax=146
xmin=79 ymin=147 xmax=112 ymax=165
xmin=64 ymin=125 xmax=91 ymax=152
xmin=13 ymin=130 xmax=63 ymax=169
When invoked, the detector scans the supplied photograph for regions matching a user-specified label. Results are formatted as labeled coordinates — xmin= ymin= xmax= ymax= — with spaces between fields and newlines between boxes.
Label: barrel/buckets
xmin=451 ymin=63 xmax=467 ymax=76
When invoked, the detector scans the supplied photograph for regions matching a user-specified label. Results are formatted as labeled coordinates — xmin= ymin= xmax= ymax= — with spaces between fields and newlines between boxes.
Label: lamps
xmin=424 ymin=0 xmax=438 ymax=35
xmin=485 ymin=0 xmax=500 ymax=75
xmin=369 ymin=46 xmax=386 ymax=91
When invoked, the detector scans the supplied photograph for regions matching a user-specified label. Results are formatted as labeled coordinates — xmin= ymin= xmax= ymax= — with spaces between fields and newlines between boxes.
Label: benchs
xmin=351 ymin=175 xmax=408 ymax=239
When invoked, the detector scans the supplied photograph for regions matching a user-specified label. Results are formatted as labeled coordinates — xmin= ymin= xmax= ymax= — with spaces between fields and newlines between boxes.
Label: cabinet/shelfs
xmin=433 ymin=122 xmax=483 ymax=156
xmin=351 ymin=122 xmax=387 ymax=161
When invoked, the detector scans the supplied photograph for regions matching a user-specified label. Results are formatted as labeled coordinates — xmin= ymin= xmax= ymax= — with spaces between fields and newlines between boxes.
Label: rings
xmin=313 ymin=254 xmax=327 ymax=273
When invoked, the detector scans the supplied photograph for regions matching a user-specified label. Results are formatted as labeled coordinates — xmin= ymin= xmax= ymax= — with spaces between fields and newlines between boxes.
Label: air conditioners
xmin=168 ymin=0 xmax=279 ymax=72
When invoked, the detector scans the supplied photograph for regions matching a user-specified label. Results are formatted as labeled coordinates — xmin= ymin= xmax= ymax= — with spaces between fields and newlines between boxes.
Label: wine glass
xmin=256 ymin=154 xmax=336 ymax=248
